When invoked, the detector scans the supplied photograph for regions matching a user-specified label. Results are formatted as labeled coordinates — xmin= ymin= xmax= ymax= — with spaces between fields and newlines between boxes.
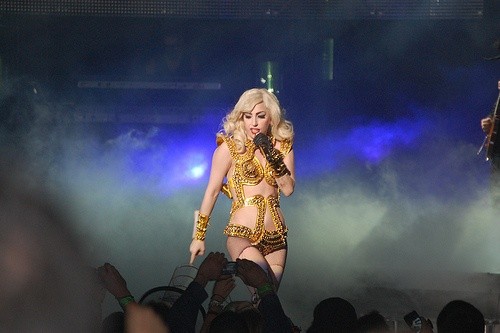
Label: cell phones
xmin=220 ymin=261 xmax=238 ymax=274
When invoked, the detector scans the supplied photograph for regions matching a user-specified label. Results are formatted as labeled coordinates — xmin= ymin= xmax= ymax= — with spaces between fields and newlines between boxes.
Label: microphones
xmin=256 ymin=132 xmax=271 ymax=162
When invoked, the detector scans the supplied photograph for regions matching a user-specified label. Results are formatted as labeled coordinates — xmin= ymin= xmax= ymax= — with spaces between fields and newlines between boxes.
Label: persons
xmin=482 ymin=81 xmax=500 ymax=193
xmin=190 ymin=89 xmax=294 ymax=307
xmin=92 ymin=252 xmax=500 ymax=333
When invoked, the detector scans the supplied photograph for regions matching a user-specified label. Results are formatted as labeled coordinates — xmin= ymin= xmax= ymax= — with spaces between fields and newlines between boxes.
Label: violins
xmin=485 ymin=113 xmax=499 ymax=161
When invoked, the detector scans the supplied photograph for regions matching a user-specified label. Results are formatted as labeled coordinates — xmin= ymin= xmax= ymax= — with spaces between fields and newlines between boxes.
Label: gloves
xmin=267 ymin=148 xmax=288 ymax=178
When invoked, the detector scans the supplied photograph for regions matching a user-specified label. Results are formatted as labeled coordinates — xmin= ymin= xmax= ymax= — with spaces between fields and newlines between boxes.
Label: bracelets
xmin=210 ymin=299 xmax=224 ymax=310
xmin=119 ymin=296 xmax=134 ymax=306
xmin=258 ymin=285 xmax=273 ymax=294
